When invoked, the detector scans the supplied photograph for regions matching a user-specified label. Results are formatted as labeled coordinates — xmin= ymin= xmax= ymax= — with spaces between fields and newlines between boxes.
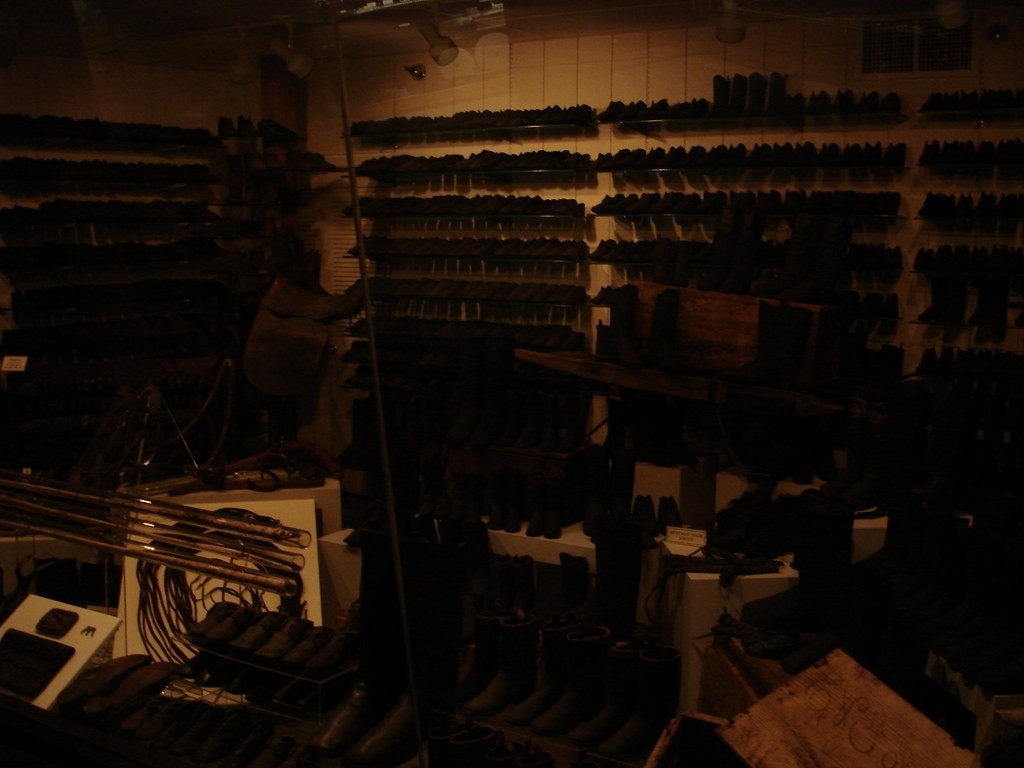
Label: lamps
xmin=418 ymin=18 xmax=460 ymax=66
xmin=714 ymin=0 xmax=747 ymax=44
xmin=269 ymin=23 xmax=312 ymax=79
xmin=934 ymin=0 xmax=970 ymax=31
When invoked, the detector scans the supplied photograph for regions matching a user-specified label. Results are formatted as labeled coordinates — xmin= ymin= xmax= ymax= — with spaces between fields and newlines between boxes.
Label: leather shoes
xmin=307 ymin=679 xmax=427 ymax=767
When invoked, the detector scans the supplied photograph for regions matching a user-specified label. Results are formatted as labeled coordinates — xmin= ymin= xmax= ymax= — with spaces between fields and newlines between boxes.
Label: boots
xmin=397 ymin=512 xmax=682 ymax=768
xmin=969 ymin=259 xmax=1009 ymax=327
xmin=747 ymin=72 xmax=788 ymax=115
xmin=592 ymin=285 xmax=680 ymax=374
xmin=739 ymin=291 xmax=901 ymax=402
xmin=784 ymin=207 xmax=860 ymax=305
xmin=710 ymin=74 xmax=747 ymax=116
xmin=918 ymin=262 xmax=963 ymax=324
xmin=692 ymin=209 xmax=772 ymax=295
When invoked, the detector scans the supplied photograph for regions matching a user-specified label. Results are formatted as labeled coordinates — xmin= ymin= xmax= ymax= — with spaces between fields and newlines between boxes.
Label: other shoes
xmin=0 ymin=116 xmax=335 ymax=392
xmin=335 ymin=366 xmax=611 ymax=539
xmin=337 ymin=105 xmax=597 ymax=366
xmin=585 ymin=390 xmax=1024 ymax=768
xmin=598 ymin=89 xmax=903 ymax=279
xmin=189 ymin=604 xmax=359 ymax=669
xmin=55 ymin=654 xmax=319 ymax=768
xmin=913 ymin=89 xmax=1024 ymax=294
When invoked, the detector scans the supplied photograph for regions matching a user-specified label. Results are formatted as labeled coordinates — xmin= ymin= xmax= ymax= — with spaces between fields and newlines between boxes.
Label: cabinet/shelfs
xmin=0 ymin=106 xmax=1024 ymax=768
